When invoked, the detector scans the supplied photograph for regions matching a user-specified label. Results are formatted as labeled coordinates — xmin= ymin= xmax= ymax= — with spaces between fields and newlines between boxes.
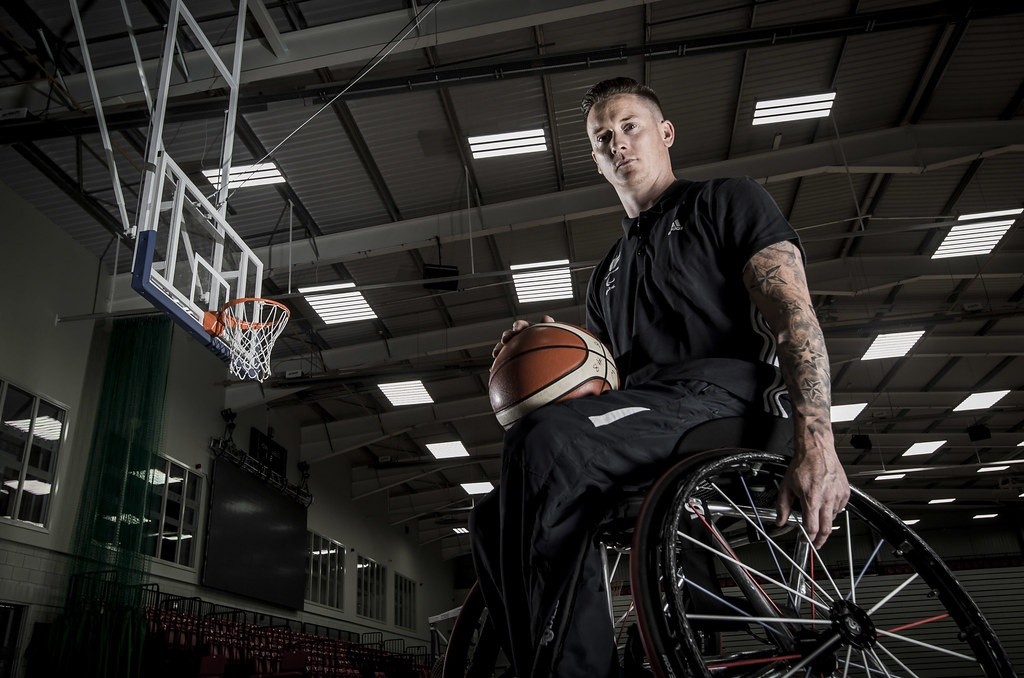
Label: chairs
xmin=145 ymin=605 xmax=432 ymax=678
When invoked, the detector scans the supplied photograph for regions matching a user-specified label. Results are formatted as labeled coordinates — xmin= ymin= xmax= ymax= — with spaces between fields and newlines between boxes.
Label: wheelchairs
xmin=441 ymin=413 xmax=1019 ymax=678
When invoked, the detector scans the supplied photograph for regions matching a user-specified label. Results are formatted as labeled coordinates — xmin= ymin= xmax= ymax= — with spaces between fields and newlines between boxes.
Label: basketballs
xmin=488 ymin=320 xmax=618 ymax=432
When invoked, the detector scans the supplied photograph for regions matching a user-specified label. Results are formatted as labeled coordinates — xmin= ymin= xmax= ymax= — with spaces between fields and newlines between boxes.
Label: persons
xmin=469 ymin=77 xmax=851 ymax=678
